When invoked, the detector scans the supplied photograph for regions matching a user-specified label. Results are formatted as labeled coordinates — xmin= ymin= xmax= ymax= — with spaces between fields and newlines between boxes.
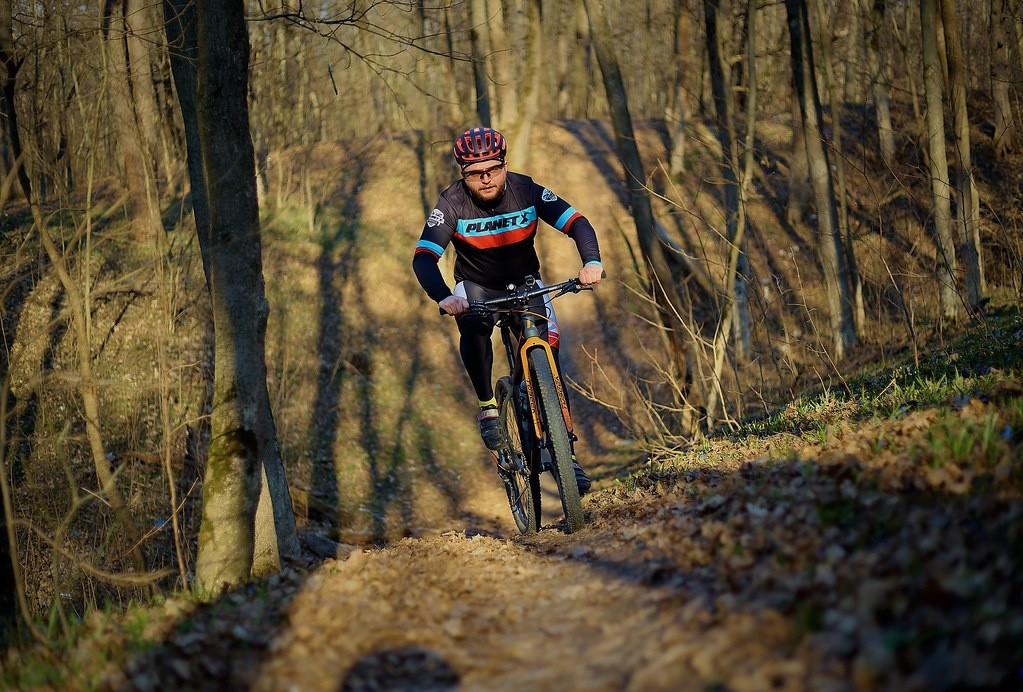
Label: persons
xmin=412 ymin=127 xmax=602 ymax=492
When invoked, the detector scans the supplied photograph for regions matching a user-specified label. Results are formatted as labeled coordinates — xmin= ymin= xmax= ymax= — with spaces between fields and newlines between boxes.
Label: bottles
xmin=519 ymin=379 xmax=528 ymax=432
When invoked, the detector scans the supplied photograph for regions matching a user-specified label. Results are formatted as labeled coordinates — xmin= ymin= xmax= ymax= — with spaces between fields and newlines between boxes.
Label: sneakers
xmin=477 ymin=403 xmax=505 ymax=450
xmin=572 ymin=461 xmax=591 ymax=491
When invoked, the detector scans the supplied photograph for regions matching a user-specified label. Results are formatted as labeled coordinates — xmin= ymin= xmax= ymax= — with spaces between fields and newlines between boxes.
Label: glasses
xmin=462 ymin=160 xmax=504 ymax=182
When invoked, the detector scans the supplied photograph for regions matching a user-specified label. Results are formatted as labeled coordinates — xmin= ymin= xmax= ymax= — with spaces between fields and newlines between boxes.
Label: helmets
xmin=453 ymin=127 xmax=507 ymax=165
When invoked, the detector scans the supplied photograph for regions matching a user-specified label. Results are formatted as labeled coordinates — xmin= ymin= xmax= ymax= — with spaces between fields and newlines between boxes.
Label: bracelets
xmin=587 ymin=260 xmax=603 ymax=267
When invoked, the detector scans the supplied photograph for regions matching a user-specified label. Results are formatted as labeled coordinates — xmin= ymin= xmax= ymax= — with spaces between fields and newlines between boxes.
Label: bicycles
xmin=437 ymin=271 xmax=605 ymax=535
